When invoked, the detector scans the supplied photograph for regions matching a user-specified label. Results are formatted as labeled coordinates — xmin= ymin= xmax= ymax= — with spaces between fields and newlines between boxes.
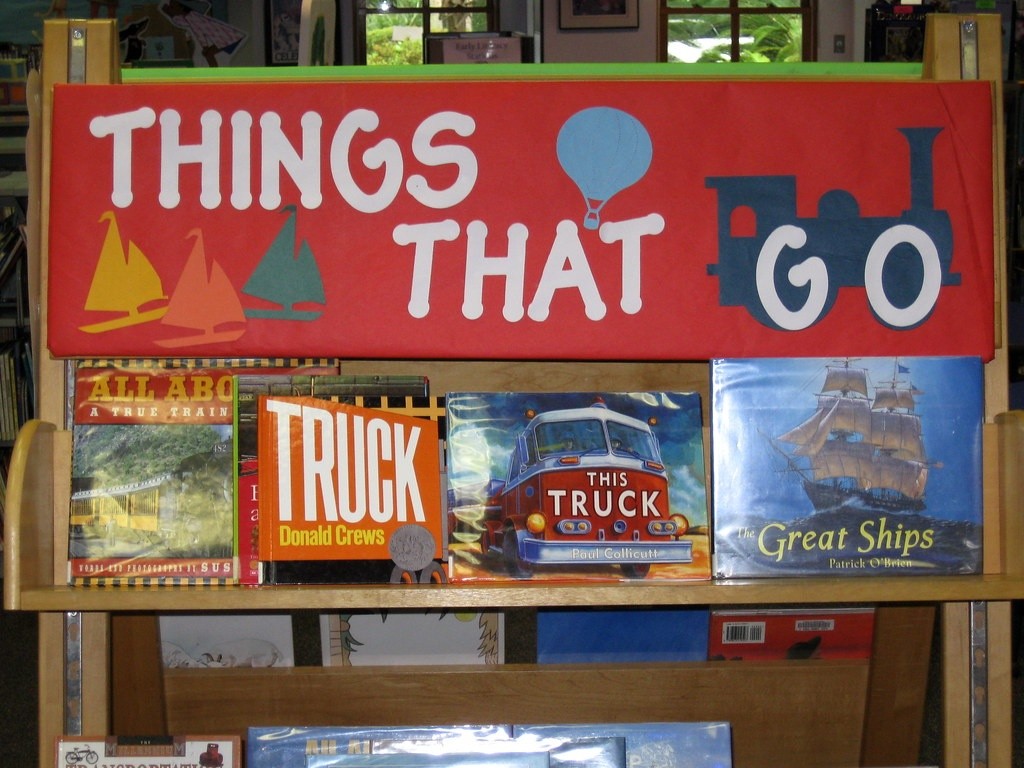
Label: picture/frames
xmin=265 ymin=0 xmax=302 ymax=66
xmin=559 ymin=0 xmax=640 ymax=29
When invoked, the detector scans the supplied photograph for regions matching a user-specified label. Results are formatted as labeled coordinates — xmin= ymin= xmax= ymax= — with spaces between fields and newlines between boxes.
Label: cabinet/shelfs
xmin=0 ymin=15 xmax=1024 ymax=767
xmin=864 ymin=8 xmax=955 ymax=62
